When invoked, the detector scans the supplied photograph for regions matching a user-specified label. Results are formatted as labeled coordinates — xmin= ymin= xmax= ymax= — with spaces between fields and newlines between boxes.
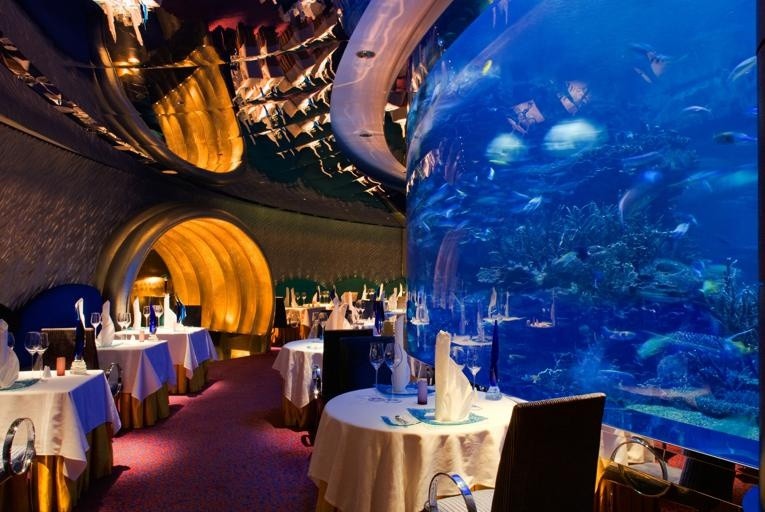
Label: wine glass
xmin=312 ymin=312 xmax=327 ymax=343
xmin=91 ymin=312 xmax=101 ymax=342
xmin=466 ymin=356 xmax=482 ymax=408
xmin=143 ymin=304 xmax=164 ymax=331
xmin=116 ymin=312 xmax=132 ymax=343
xmin=24 ymin=330 xmax=49 ymax=381
xmin=367 ymin=342 xmax=386 ymax=403
xmin=380 ymin=341 xmax=403 ymax=404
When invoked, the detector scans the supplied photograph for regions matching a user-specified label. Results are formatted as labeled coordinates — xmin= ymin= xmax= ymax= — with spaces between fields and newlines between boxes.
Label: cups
xmin=417 ymin=378 xmax=428 ymax=404
xmin=56 ymin=356 xmax=66 ymax=377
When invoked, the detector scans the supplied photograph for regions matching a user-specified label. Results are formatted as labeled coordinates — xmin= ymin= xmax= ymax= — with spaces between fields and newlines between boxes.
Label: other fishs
xmin=406 ymin=41 xmax=759 ymax=408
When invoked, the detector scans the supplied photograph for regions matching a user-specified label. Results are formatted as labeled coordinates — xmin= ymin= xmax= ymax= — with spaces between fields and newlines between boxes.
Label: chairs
xmin=272 ymin=283 xmax=605 ymax=511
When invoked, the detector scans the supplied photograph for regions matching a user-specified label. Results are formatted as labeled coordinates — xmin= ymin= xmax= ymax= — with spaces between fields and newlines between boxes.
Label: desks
xmin=0 ymin=282 xmax=219 ymax=512
